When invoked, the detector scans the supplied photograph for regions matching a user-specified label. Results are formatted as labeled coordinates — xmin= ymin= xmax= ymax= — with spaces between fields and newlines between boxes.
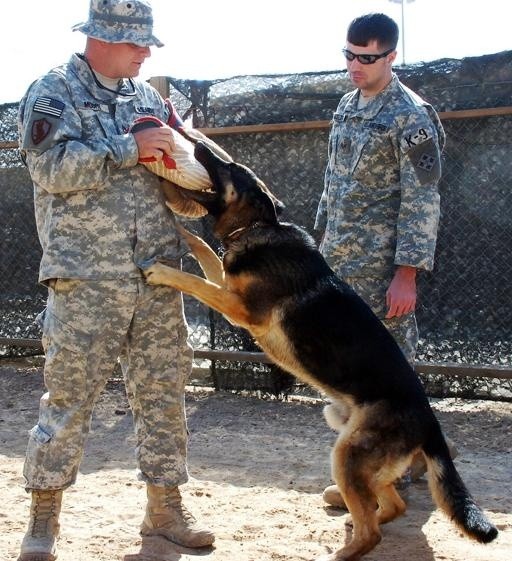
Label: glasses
xmin=341 ymin=48 xmax=396 ymax=64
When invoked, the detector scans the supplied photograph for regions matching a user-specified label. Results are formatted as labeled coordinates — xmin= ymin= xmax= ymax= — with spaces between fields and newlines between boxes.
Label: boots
xmin=22 ymin=489 xmax=61 ymax=559
xmin=411 ymin=432 xmax=458 ymax=479
xmin=142 ymin=485 xmax=214 ymax=548
xmin=324 ymin=483 xmax=344 ymax=506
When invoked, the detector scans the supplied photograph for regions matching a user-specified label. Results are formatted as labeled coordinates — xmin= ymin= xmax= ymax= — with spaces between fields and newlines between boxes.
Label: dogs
xmin=142 ymin=142 xmax=499 ymax=560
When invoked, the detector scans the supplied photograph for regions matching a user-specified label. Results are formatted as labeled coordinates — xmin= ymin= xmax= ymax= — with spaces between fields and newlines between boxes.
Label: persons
xmin=17 ymin=1 xmax=216 ymax=561
xmin=315 ymin=13 xmax=458 ymax=510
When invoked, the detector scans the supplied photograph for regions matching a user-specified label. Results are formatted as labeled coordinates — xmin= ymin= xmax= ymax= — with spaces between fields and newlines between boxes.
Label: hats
xmin=71 ymin=0 xmax=164 ymax=47
